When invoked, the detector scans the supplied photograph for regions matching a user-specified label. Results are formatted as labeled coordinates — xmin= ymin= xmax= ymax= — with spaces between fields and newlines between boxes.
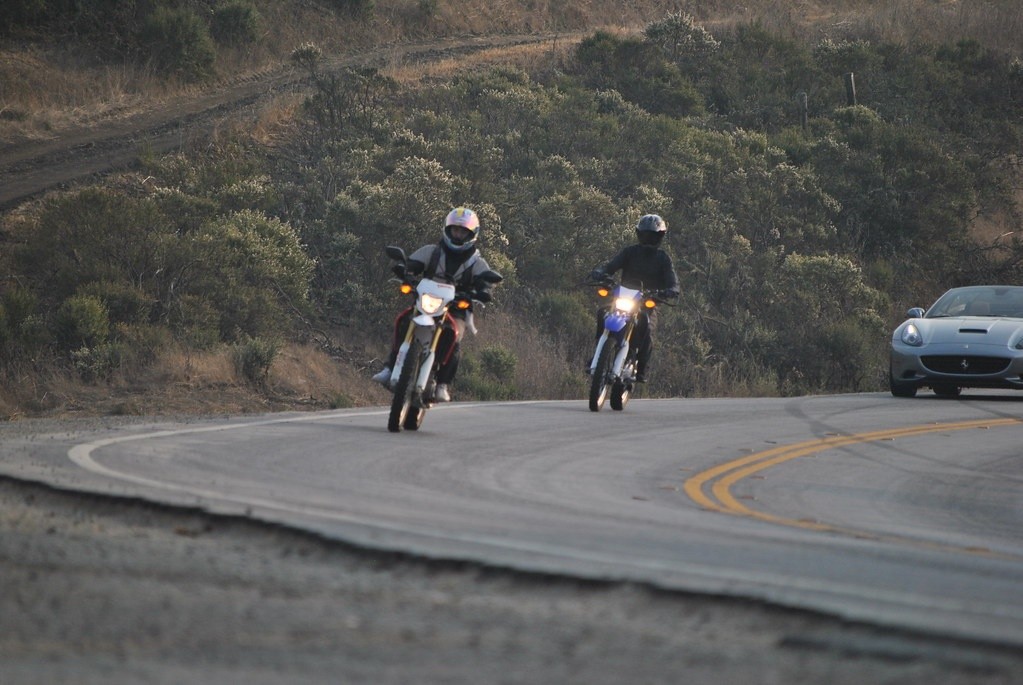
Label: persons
xmin=585 ymin=213 xmax=680 ymax=383
xmin=370 ymin=208 xmax=493 ymax=401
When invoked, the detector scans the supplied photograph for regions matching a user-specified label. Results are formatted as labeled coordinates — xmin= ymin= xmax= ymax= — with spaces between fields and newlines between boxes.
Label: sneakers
xmin=435 ymin=383 xmax=451 ymax=402
xmin=371 ymin=366 xmax=392 ymax=384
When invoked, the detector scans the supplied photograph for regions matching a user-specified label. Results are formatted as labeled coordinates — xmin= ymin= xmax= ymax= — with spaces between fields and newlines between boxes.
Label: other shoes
xmin=636 ymin=362 xmax=648 ymax=383
xmin=587 ymin=355 xmax=595 ymax=367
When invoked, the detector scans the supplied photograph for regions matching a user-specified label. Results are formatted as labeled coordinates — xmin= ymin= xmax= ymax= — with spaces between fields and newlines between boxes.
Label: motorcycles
xmin=382 ymin=245 xmax=504 ymax=435
xmin=584 ymin=270 xmax=676 ymax=411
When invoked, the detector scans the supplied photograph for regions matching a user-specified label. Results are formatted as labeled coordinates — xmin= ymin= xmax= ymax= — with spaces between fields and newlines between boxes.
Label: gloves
xmin=592 ymin=268 xmax=605 ymax=279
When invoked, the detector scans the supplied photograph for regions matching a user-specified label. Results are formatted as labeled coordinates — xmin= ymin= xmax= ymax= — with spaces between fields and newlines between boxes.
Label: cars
xmin=889 ymin=285 xmax=1022 ymax=399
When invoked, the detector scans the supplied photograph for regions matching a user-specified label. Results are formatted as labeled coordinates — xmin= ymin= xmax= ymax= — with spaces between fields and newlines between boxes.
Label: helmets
xmin=442 ymin=206 xmax=480 ymax=251
xmin=635 ymin=213 xmax=666 ymax=233
